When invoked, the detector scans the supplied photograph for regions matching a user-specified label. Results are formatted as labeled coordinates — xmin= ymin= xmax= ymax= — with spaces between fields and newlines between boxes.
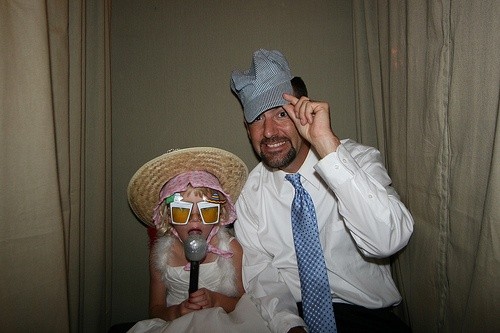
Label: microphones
xmin=184 ymin=234 xmax=207 ymax=303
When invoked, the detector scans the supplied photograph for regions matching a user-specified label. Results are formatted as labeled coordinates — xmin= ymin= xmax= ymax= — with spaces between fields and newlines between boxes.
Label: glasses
xmin=170 ymin=193 xmax=227 ymax=225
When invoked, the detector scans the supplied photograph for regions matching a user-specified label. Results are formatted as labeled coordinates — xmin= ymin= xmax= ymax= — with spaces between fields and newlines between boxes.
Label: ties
xmin=284 ymin=172 xmax=337 ymax=333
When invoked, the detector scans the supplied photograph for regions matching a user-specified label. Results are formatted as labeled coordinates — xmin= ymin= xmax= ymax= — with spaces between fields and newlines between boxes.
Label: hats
xmin=230 ymin=48 xmax=295 ymax=124
xmin=127 ymin=147 xmax=249 ymax=228
xmin=152 ymin=171 xmax=238 ymax=232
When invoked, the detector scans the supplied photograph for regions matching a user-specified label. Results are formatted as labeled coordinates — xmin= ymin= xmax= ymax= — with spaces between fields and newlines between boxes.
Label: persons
xmin=148 ymin=170 xmax=245 ymax=333
xmin=233 ymin=75 xmax=415 ymax=333
